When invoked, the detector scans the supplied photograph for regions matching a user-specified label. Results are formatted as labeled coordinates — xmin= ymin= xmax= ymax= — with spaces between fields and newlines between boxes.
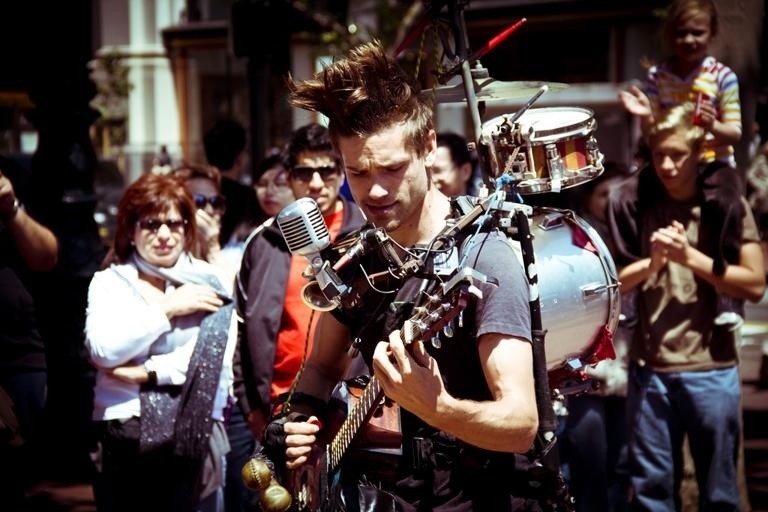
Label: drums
xmin=505 ymin=206 xmax=622 ymax=401
xmin=480 ymin=106 xmax=606 ymax=196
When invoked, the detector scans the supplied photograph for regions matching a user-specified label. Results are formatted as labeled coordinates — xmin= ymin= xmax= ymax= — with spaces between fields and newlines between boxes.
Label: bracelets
xmin=10 ymin=197 xmax=19 ymax=215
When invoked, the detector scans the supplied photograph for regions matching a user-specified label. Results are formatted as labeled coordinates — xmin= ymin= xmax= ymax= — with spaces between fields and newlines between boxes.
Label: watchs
xmin=144 ymin=358 xmax=157 ymax=384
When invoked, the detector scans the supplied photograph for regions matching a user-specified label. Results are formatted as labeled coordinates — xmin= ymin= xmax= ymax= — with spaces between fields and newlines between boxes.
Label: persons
xmin=262 ymin=37 xmax=541 ymax=512
xmin=432 ymin=0 xmax=768 ymax=512
xmin=80 ymin=120 xmax=372 ymax=512
xmin=0 ymin=154 xmax=60 ymax=414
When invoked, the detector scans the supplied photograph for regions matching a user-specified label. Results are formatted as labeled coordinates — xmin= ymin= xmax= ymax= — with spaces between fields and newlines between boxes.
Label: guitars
xmin=286 ymin=266 xmax=487 ymax=512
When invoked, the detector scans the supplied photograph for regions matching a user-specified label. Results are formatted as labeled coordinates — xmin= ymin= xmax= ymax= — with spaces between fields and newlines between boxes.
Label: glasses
xmin=133 ymin=217 xmax=189 ymax=234
xmin=191 ymin=192 xmax=228 ymax=217
xmin=286 ymin=165 xmax=339 ymax=183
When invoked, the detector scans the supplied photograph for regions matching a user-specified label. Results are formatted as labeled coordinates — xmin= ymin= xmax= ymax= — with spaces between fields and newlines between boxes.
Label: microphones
xmin=277 ymin=197 xmax=330 ymax=258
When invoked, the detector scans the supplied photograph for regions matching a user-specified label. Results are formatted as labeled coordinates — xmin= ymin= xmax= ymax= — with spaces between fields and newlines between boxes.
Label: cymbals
xmin=420 ymin=77 xmax=571 ymax=102
